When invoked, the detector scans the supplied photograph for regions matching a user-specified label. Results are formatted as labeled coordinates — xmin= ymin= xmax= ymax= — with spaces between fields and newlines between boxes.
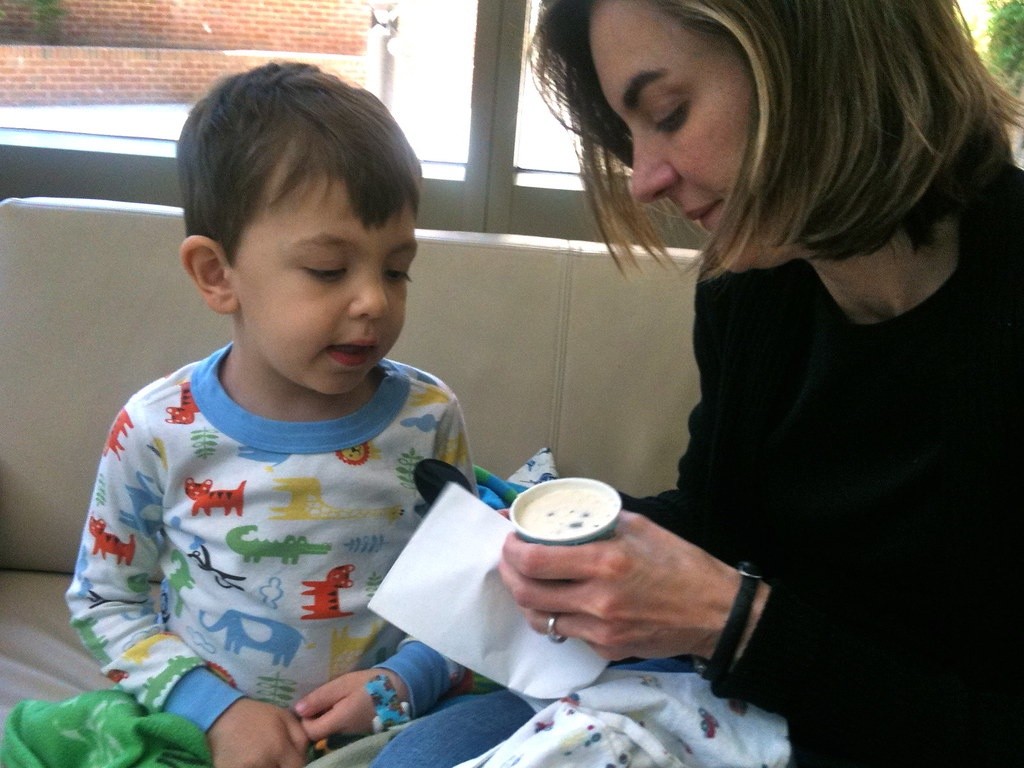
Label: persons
xmin=375 ymin=0 xmax=1024 ymax=768
xmin=63 ymin=58 xmax=479 ymax=768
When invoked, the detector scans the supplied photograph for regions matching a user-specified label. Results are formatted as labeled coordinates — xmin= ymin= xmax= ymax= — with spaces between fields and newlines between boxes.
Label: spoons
xmin=412 ymin=456 xmax=477 ymax=508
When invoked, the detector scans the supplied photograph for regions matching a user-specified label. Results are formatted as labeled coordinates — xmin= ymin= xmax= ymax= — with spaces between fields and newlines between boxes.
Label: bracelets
xmin=691 ymin=560 xmax=761 ymax=680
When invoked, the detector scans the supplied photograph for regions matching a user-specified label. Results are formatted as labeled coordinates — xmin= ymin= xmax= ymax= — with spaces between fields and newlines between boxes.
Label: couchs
xmin=0 ymin=196 xmax=700 ymax=728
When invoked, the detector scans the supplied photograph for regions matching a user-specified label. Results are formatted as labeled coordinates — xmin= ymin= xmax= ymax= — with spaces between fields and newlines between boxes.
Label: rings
xmin=546 ymin=613 xmax=568 ymax=644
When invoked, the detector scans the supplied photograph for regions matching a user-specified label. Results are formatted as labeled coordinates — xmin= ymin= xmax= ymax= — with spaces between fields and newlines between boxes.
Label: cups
xmin=508 ymin=477 xmax=623 ymax=546
